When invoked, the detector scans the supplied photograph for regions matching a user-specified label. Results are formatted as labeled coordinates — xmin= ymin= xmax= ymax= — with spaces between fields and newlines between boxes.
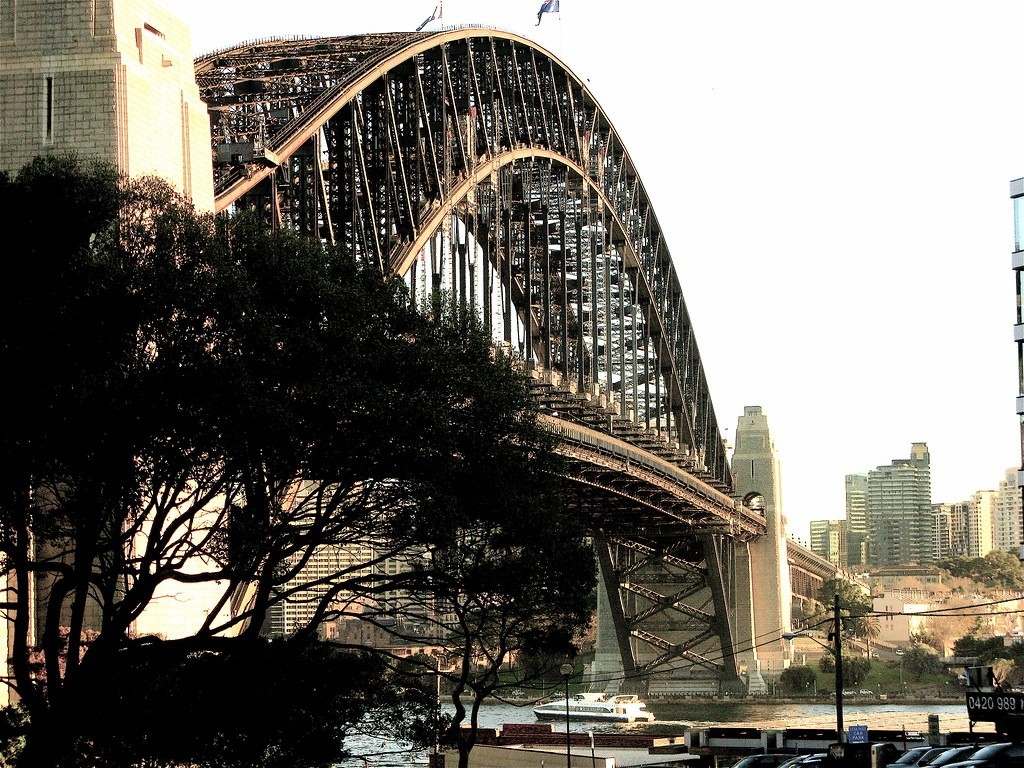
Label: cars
xmin=777 ymin=753 xmax=828 ymax=768
xmin=918 ymin=745 xmax=984 ymax=768
xmin=886 ymin=746 xmax=952 ymax=768
xmin=731 ymin=754 xmax=794 ymax=768
xmin=860 ymin=689 xmax=873 ymax=694
xmin=940 ymin=742 xmax=1024 ymax=768
xmin=896 ymin=650 xmax=904 ymax=656
xmin=842 ymin=689 xmax=853 ymax=695
xmin=550 ymin=692 xmax=562 ymax=699
xmin=512 ymin=690 xmax=525 ymax=696
xmin=873 ymin=653 xmax=879 ymax=658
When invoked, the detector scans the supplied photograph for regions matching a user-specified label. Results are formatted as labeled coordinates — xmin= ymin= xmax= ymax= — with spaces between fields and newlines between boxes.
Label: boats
xmin=531 ymin=693 xmax=655 ymax=724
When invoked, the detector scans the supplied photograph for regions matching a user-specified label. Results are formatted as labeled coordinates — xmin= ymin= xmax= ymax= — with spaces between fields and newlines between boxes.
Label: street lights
xmin=560 ymin=664 xmax=573 ymax=768
xmin=782 ymin=632 xmax=843 ymax=743
